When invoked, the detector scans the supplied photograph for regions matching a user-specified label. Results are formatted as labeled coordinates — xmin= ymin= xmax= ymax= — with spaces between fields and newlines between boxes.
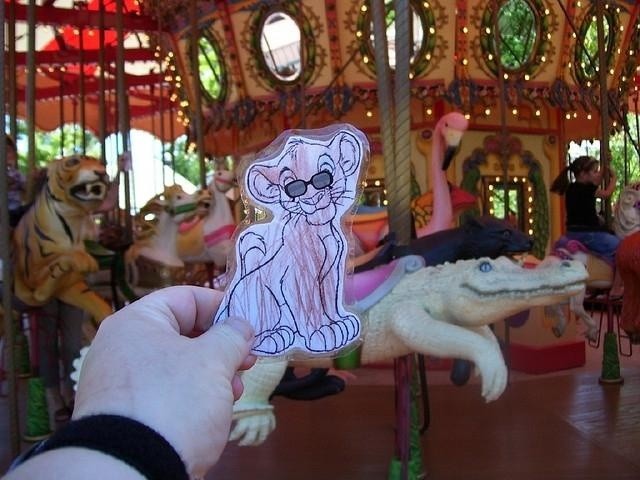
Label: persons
xmin=0 ymin=271 xmax=259 ymax=480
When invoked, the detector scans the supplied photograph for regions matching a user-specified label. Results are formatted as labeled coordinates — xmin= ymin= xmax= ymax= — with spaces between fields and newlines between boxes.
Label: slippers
xmin=609 ymin=289 xmax=625 ymax=301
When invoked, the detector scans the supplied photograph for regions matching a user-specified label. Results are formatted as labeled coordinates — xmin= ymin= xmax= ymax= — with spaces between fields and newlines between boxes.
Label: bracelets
xmin=7 ymin=413 xmax=195 ymax=480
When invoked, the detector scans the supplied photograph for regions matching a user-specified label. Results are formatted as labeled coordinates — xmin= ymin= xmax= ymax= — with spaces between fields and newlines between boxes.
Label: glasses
xmin=284 ymin=170 xmax=334 ymax=202
